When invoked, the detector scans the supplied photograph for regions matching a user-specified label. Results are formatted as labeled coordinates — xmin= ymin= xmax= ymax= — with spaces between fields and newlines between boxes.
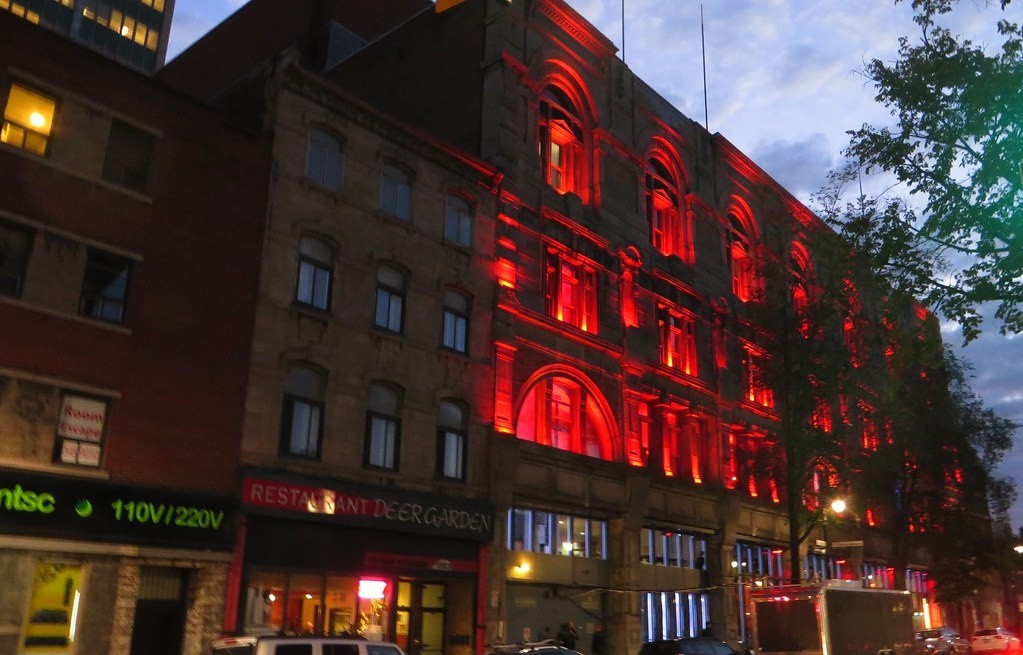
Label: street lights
xmin=821 ymin=497 xmax=849 ymax=578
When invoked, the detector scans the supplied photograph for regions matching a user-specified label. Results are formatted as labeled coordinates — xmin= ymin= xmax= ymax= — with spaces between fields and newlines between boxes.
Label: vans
xmin=210 ymin=636 xmax=405 ymax=655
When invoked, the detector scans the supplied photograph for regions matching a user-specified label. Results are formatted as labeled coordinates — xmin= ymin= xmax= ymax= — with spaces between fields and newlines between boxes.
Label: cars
xmin=915 ymin=628 xmax=1023 ymax=655
xmin=636 ymin=636 xmax=738 ymax=655
xmin=519 ymin=646 xmax=584 ymax=655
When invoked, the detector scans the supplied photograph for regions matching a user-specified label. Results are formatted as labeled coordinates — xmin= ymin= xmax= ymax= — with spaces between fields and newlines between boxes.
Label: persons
xmin=556 ymin=620 xmax=579 ymax=651
xmin=702 ymin=622 xmax=712 ymax=637
xmin=696 ymin=550 xmax=704 ymax=569
xmin=591 ymin=624 xmax=607 ymax=655
